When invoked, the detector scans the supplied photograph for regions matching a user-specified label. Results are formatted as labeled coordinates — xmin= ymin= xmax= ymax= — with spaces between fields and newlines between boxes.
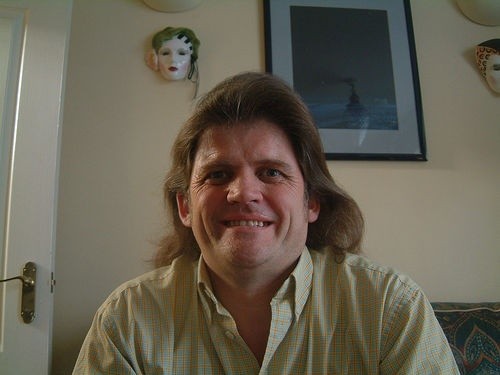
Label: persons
xmin=475 ymin=38 xmax=500 ymax=94
xmin=73 ymin=73 xmax=462 ymax=374
xmin=145 ymin=26 xmax=200 ymax=80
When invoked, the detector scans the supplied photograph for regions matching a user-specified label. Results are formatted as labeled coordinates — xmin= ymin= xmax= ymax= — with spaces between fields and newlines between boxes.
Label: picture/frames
xmin=263 ymin=0 xmax=427 ymax=162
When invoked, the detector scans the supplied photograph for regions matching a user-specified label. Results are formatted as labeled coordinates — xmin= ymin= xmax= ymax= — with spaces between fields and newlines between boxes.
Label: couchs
xmin=430 ymin=301 xmax=500 ymax=375
